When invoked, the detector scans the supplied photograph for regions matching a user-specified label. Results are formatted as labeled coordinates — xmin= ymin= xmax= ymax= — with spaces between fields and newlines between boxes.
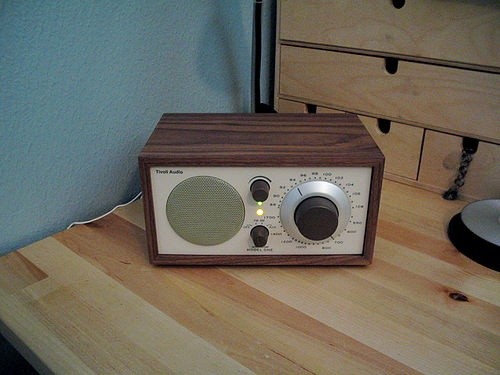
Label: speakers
xmin=138 ymin=112 xmax=386 ymax=269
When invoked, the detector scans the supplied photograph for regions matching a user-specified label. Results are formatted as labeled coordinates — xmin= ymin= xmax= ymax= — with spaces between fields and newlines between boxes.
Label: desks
xmin=1 ymin=179 xmax=500 ymax=373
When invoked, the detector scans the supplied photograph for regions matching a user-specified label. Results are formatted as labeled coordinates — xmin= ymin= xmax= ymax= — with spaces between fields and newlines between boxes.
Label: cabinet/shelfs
xmin=274 ymin=0 xmax=500 ymax=200
